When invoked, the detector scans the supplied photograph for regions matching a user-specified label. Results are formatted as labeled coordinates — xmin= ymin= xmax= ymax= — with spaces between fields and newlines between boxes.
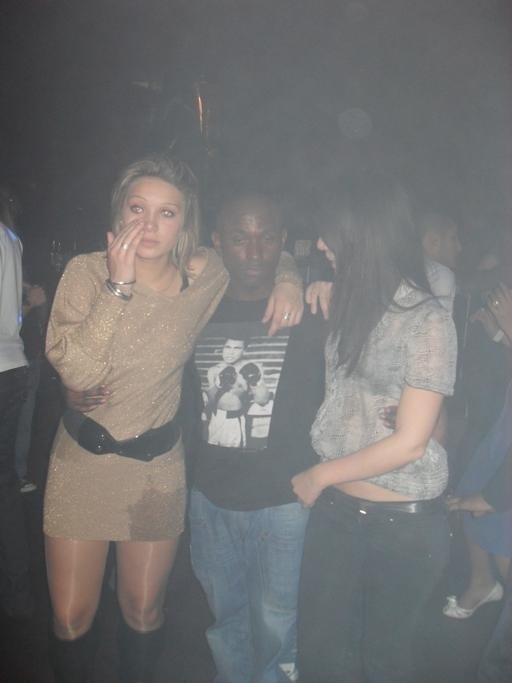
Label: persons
xmin=293 ymin=176 xmax=457 ymax=683
xmin=186 ymin=191 xmax=329 ymax=682
xmin=39 ymin=148 xmax=306 ymax=683
xmin=5 ymin=197 xmax=512 ymax=624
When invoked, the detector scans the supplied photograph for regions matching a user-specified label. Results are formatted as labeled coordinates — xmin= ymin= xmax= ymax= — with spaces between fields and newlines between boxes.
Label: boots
xmin=118 ymin=613 xmax=165 ymax=682
xmin=47 ymin=616 xmax=98 ymax=683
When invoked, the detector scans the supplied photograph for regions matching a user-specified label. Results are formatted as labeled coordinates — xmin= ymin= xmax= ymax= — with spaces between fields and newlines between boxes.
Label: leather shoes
xmin=441 ymin=579 xmax=504 ymax=619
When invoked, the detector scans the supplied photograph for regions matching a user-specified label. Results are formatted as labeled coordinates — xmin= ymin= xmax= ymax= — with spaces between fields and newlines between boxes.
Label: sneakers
xmin=20 ymin=478 xmax=37 ymax=493
